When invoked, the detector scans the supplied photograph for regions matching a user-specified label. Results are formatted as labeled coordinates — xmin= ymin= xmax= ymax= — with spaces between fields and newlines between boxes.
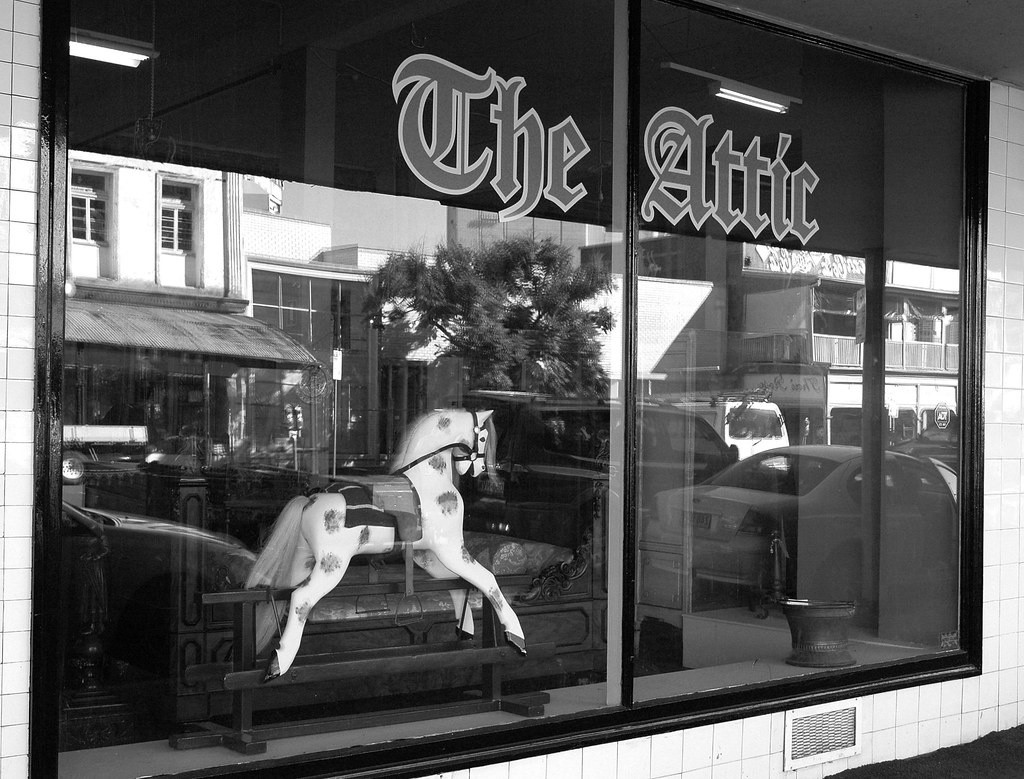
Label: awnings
xmin=65 ymin=299 xmax=322 ymax=363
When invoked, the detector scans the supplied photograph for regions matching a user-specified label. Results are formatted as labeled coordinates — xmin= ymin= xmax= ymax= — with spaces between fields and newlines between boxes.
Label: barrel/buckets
xmin=780 ymin=599 xmax=856 ymax=668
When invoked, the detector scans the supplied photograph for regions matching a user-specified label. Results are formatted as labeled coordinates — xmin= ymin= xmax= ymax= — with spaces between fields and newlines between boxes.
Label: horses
xmin=239 ymin=409 xmax=528 ymax=682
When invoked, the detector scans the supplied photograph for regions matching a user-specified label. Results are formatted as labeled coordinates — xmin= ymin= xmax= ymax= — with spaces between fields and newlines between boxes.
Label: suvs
xmin=482 ymin=394 xmax=745 ymax=578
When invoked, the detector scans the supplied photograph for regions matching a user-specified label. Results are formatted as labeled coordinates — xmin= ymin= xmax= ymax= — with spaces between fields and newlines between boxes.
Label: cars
xmin=645 ymin=444 xmax=959 ymax=607
xmin=60 ymin=495 xmax=259 ymax=690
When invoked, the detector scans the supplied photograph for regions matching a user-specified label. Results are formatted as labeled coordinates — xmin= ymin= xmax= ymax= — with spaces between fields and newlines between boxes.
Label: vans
xmin=670 ymin=400 xmax=790 ymax=473
